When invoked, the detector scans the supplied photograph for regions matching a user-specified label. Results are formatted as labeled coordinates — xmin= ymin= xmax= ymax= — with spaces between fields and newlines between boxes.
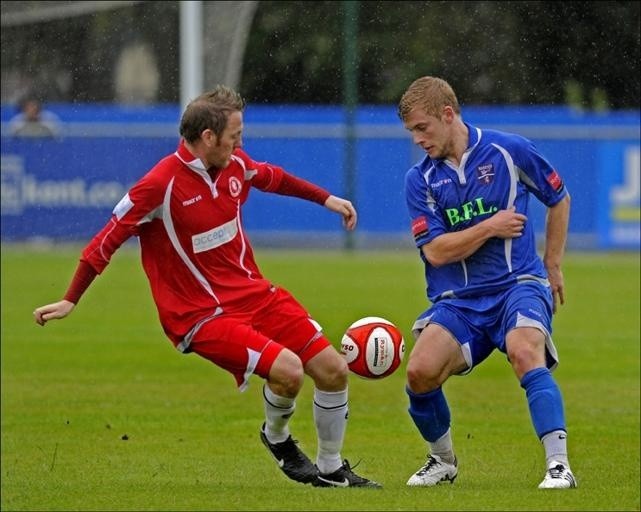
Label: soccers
xmin=340 ymin=315 xmax=406 ymax=381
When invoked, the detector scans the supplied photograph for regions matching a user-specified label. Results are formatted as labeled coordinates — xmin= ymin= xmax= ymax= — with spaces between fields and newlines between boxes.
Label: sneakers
xmin=260 ymin=421 xmax=320 ymax=484
xmin=538 ymin=460 xmax=578 ymax=490
xmin=312 ymin=459 xmax=383 ymax=490
xmin=404 ymin=453 xmax=458 ymax=487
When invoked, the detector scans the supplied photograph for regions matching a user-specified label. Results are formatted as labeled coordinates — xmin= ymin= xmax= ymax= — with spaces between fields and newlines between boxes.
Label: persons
xmin=396 ymin=72 xmax=579 ymax=491
xmin=30 ymin=81 xmax=384 ymax=492
xmin=5 ymin=90 xmax=65 ymax=126
